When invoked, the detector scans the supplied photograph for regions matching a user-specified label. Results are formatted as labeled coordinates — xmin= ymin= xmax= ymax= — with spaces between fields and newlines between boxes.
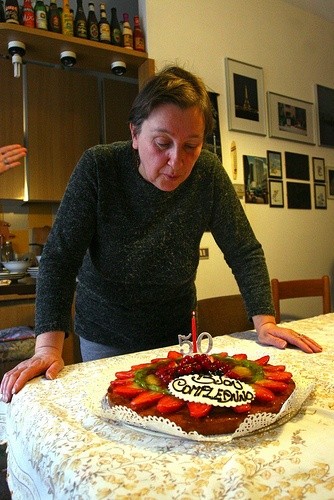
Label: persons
xmin=0 ymin=64 xmax=324 ymax=404
xmin=0 ymin=144 xmax=28 ymax=175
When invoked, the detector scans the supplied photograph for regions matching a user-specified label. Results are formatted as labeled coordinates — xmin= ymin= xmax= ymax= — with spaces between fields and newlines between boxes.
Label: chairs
xmin=194 ymin=294 xmax=251 ymax=339
xmin=271 ymin=274 xmax=331 ymax=322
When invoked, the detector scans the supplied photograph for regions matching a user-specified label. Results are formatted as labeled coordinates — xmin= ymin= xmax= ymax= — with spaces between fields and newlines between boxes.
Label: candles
xmin=191 ymin=311 xmax=197 ymax=352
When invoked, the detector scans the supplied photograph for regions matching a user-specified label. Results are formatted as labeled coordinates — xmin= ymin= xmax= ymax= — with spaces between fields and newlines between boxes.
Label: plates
xmin=121 ymin=408 xmax=300 ymax=441
xmin=27 ymin=267 xmax=39 ymax=278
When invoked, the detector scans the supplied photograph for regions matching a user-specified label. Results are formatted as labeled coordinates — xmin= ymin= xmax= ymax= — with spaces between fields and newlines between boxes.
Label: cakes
xmin=106 ymin=333 xmax=296 ymax=436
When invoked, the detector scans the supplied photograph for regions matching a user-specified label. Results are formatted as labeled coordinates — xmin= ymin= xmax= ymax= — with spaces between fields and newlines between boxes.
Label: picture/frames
xmin=267 ymin=149 xmax=283 ymax=177
xmin=313 ymin=156 xmax=324 ymax=181
xmin=269 ymin=180 xmax=285 ymax=207
xmin=313 ymin=184 xmax=328 ymax=209
xmin=266 ymin=90 xmax=316 ymax=144
xmin=227 ymin=57 xmax=268 ymax=137
xmin=327 ymin=168 xmax=334 ymax=199
xmin=313 ymin=83 xmax=334 ymax=148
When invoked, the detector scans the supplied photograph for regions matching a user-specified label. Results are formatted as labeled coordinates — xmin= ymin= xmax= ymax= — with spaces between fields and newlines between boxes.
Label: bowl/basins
xmin=2 ymin=261 xmax=29 ymax=274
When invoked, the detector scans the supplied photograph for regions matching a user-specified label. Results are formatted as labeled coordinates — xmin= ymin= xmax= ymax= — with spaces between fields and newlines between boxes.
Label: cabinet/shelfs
xmin=0 ymin=56 xmax=101 ymax=202
xmin=102 ymin=78 xmax=139 ymax=144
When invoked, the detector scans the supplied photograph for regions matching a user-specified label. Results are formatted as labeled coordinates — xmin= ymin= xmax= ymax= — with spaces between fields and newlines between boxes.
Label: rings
xmin=5 ymin=159 xmax=8 ymax=164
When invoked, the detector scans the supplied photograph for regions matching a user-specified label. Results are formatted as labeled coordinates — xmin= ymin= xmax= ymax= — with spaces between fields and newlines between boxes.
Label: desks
xmin=0 ymin=313 xmax=334 ymax=500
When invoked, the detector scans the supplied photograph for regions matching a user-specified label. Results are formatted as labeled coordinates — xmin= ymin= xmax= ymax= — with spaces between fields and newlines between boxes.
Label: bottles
xmin=2 ymin=240 xmax=14 ymax=262
xmin=0 ymin=0 xmax=145 ymax=52
